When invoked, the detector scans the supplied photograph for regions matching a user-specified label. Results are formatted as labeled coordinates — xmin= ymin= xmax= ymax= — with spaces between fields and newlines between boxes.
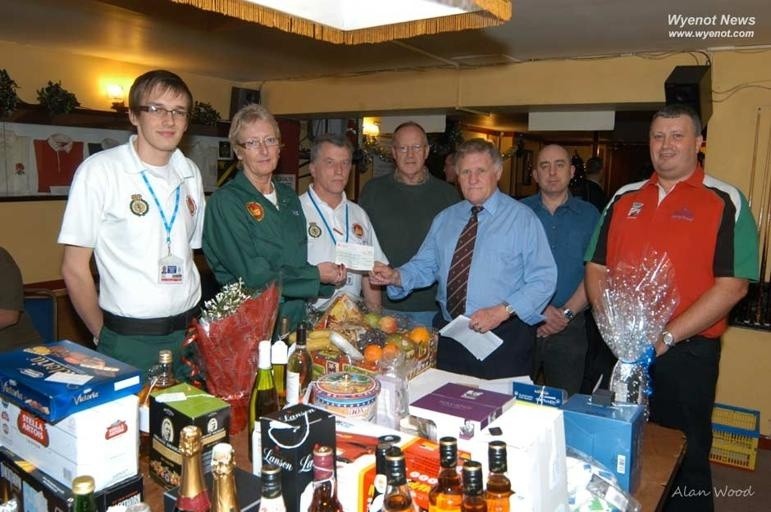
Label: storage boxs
xmin=334 ymin=410 xmax=472 ymax=512
xmin=1 ymin=339 xmax=337 ymax=512
xmin=561 ymin=391 xmax=644 ymax=498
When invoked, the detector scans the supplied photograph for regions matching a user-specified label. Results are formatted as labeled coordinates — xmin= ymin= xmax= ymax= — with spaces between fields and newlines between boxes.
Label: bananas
xmin=288 ymin=329 xmax=353 ymax=353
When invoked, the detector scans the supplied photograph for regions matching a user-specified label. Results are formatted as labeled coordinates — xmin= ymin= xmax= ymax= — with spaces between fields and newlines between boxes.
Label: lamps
xmin=173 ymin=0 xmax=513 ymax=51
xmin=104 ymin=82 xmax=131 ymax=115
xmin=363 ymin=122 xmax=381 ymax=154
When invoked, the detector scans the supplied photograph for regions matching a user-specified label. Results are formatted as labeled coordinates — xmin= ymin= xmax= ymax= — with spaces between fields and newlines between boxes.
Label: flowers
xmin=201 ymin=279 xmax=254 ymax=324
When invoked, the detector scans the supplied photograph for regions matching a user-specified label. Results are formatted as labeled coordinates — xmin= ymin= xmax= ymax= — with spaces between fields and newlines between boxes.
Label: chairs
xmin=21 ymin=288 xmax=59 ymax=345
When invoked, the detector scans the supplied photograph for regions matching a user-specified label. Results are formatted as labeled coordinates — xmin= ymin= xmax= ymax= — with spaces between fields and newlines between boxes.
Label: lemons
xmin=364 ymin=313 xmax=380 ymax=330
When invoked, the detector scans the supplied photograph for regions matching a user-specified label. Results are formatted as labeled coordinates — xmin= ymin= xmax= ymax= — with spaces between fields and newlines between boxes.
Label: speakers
xmin=230 ymin=87 xmax=260 ymax=121
xmin=665 ymin=65 xmax=713 ymax=130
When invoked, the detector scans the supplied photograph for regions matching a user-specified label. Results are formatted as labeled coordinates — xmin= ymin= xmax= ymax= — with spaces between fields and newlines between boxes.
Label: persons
xmin=515 ymin=144 xmax=602 ymax=397
xmin=581 ymin=102 xmax=761 ymax=512
xmin=443 ymin=153 xmax=457 ymax=182
xmin=367 ymin=137 xmax=558 ymax=381
xmin=357 ymin=121 xmax=460 ymax=330
xmin=56 ymin=70 xmax=211 ymax=382
xmin=201 ymin=104 xmax=347 ymax=346
xmin=571 ymin=156 xmax=610 ymax=214
xmin=298 ymin=132 xmax=390 ymax=312
xmin=0 ymin=247 xmax=45 ymax=352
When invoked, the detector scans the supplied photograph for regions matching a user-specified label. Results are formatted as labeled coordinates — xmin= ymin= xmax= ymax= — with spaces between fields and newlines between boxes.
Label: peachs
xmin=379 ymin=316 xmax=399 ymax=333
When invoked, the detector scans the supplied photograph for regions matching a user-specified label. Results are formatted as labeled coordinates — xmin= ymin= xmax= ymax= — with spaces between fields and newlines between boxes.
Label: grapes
xmin=357 ymin=329 xmax=385 ymax=351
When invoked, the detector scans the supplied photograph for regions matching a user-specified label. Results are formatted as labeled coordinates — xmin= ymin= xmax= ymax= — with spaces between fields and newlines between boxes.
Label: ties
xmin=445 ymin=205 xmax=484 ymax=320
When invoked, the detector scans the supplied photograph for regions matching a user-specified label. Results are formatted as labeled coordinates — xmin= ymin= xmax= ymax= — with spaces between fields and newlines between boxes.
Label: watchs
xmin=662 ymin=330 xmax=676 ymax=349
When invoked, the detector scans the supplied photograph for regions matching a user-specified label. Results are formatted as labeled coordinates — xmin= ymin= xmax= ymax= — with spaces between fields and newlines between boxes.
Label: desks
xmin=140 ymin=419 xmax=691 ymax=512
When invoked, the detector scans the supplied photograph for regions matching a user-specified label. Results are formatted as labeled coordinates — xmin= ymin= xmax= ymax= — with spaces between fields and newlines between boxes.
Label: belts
xmin=676 ymin=333 xmax=720 ymax=343
xmin=105 ymin=300 xmax=205 ymax=336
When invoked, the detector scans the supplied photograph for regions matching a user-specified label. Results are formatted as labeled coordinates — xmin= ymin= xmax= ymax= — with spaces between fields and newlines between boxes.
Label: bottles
xmin=69 ymin=319 xmax=514 ymax=512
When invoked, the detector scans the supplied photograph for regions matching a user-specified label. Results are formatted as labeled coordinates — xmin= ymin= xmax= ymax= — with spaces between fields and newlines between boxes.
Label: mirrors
xmin=273 ymin=100 xmax=705 ymax=207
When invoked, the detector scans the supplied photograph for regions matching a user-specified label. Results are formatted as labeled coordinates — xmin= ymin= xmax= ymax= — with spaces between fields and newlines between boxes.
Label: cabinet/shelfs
xmin=0 ymin=98 xmax=238 ymax=202
xmin=22 ymin=272 xmax=103 ymax=350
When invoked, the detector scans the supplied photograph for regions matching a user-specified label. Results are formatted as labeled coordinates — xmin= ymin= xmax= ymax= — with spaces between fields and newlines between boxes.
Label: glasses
xmin=138 ymin=105 xmax=190 ymax=122
xmin=396 ymin=144 xmax=426 ymax=152
xmin=235 ymin=137 xmax=281 ymax=149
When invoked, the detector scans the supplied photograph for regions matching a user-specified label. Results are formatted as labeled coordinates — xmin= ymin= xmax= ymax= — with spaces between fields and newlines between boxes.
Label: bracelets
xmin=561 ymin=305 xmax=576 ymax=320
xmin=502 ymin=301 xmax=516 ymax=317
